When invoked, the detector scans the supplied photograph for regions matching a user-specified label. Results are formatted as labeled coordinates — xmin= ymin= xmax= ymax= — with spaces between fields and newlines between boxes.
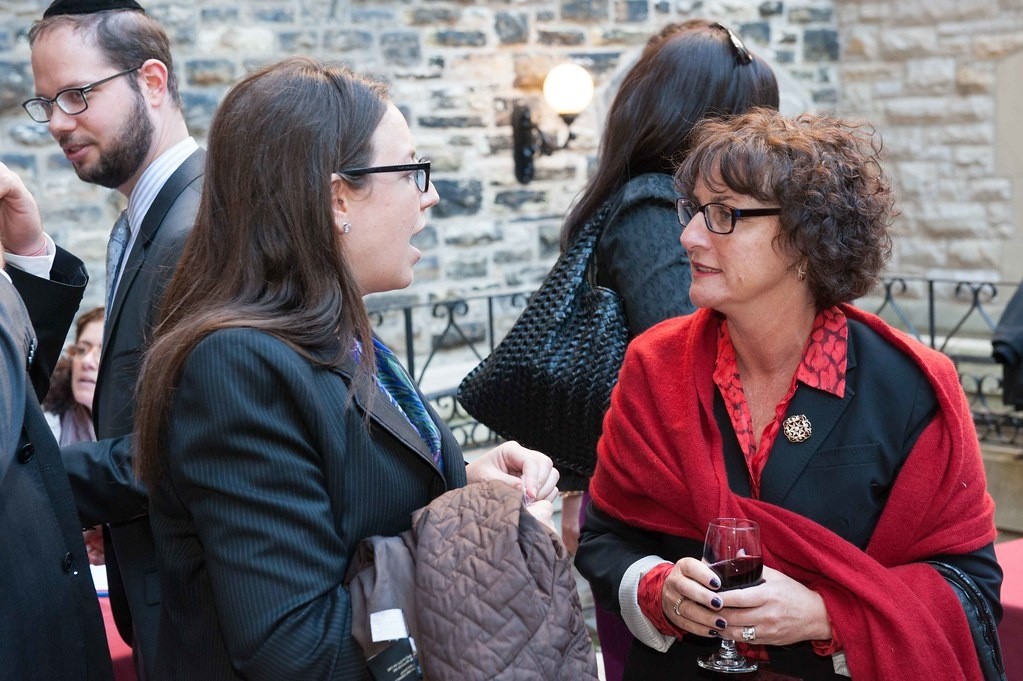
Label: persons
xmin=0 ymin=2 xmax=1006 ymax=681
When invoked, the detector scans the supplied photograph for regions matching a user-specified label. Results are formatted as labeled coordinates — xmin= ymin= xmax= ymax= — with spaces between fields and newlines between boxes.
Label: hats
xmin=43 ymin=0 xmax=146 ymax=18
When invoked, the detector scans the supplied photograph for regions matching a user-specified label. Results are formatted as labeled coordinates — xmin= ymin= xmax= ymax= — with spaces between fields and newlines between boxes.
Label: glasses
xmin=21 ymin=65 xmax=146 ymax=124
xmin=673 ymin=197 xmax=785 ymax=235
xmin=342 ymin=155 xmax=432 ymax=194
xmin=709 ymin=21 xmax=755 ymax=68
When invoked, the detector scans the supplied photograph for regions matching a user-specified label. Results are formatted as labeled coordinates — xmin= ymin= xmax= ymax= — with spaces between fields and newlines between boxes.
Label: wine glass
xmin=698 ymin=517 xmax=764 ymax=672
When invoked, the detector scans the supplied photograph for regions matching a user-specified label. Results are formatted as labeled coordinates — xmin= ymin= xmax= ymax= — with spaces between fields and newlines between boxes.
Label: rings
xmin=744 ymin=626 xmax=756 ymax=640
xmin=673 ymin=597 xmax=690 ymax=617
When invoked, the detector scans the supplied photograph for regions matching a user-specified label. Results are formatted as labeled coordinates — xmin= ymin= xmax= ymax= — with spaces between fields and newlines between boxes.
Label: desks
xmin=996 ymin=535 xmax=1023 ymax=681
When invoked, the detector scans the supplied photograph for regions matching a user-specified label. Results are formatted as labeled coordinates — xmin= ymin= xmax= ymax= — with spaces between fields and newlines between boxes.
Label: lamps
xmin=513 ymin=63 xmax=595 ymax=184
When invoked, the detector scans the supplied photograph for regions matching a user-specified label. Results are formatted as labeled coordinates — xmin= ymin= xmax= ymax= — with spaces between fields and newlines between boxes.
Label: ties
xmin=102 ymin=207 xmax=132 ymax=349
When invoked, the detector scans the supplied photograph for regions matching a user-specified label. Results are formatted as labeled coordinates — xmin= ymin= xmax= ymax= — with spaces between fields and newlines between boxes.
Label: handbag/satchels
xmin=456 ymin=183 xmax=630 ymax=476
